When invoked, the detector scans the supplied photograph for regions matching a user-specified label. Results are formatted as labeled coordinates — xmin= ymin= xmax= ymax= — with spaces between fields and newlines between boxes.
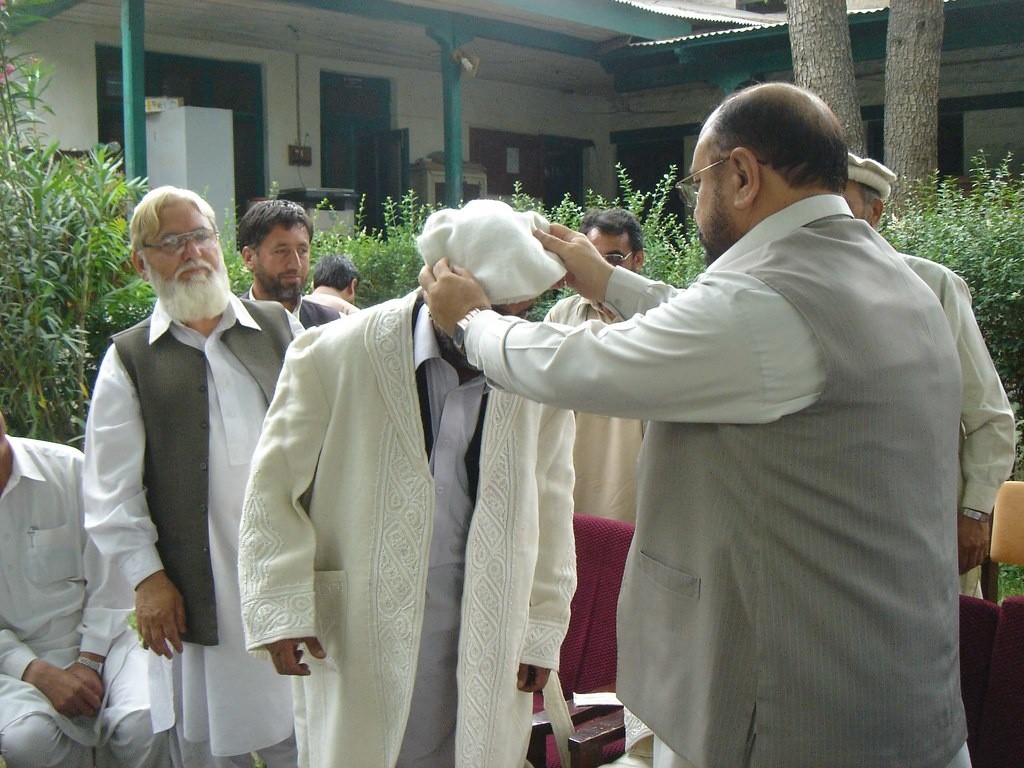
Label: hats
xmin=416 ymin=199 xmax=570 ymax=306
xmin=847 ymin=151 xmax=897 ymax=199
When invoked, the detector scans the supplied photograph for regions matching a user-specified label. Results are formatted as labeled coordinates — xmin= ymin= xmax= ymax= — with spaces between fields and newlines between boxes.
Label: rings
xmin=563 ymin=276 xmax=567 ymax=285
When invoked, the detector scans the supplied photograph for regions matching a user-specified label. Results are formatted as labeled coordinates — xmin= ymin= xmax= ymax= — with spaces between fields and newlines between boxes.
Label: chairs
xmin=533 ymin=481 xmax=1024 ymax=768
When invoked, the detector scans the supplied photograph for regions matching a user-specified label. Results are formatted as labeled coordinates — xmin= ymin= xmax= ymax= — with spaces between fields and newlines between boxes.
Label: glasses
xmin=144 ymin=228 xmax=220 ymax=257
xmin=598 ymin=251 xmax=633 ymax=268
xmin=675 ymin=155 xmax=767 ymax=209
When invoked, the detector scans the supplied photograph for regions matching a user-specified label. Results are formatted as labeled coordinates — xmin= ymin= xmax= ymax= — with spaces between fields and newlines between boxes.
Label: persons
xmin=845 ymin=152 xmax=1017 ymax=598
xmin=303 ymin=253 xmax=362 ymax=315
xmin=78 ymin=185 xmax=307 ymax=767
xmin=545 ymin=205 xmax=649 ymax=528
xmin=238 ymin=199 xmax=578 ymax=767
xmin=0 ymin=409 xmax=171 ymax=768
xmin=238 ymin=199 xmax=339 ymax=330
xmin=417 ymin=81 xmax=974 ymax=768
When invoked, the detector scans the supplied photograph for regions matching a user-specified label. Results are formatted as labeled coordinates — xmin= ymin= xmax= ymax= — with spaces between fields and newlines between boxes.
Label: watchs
xmin=453 ymin=307 xmax=488 ymax=356
xmin=77 ymin=656 xmax=104 ymax=676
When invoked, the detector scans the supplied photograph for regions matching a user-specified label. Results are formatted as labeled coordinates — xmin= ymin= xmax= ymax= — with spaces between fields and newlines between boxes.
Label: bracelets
xmin=961 ymin=507 xmax=990 ymax=522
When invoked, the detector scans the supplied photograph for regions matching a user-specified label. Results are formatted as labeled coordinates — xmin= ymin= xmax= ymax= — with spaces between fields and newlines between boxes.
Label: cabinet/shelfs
xmin=409 ymin=171 xmax=488 ymax=215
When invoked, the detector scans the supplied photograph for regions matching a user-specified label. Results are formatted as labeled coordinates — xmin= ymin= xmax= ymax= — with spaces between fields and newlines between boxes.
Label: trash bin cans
xmin=278 ymin=186 xmax=357 ymax=240
